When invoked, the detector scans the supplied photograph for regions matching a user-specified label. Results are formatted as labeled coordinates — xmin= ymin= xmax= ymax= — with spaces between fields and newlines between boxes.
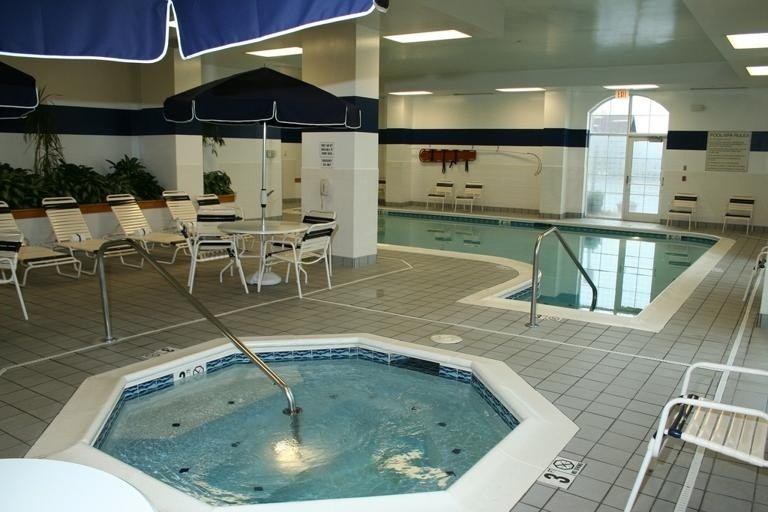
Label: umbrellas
xmin=0 ymin=60 xmax=39 ymax=120
xmin=162 ymin=67 xmax=364 ymax=275
xmin=0 ymin=0 xmax=390 ymax=64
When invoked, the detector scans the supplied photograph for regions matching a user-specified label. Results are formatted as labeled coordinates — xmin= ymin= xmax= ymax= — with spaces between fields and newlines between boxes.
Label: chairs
xmin=426 ymin=180 xmax=484 ymax=214
xmin=42 ymin=197 xmax=145 ymax=275
xmin=662 ymin=191 xmax=699 ymax=232
xmin=163 ymin=191 xmax=337 ymax=299
xmin=625 ymin=360 xmax=768 ymax=512
xmin=1 ymin=201 xmax=81 ymax=286
xmin=107 ymin=194 xmax=192 ymax=264
xmin=720 ymin=193 xmax=756 ymax=238
xmin=1 ymin=229 xmax=28 ymax=320
